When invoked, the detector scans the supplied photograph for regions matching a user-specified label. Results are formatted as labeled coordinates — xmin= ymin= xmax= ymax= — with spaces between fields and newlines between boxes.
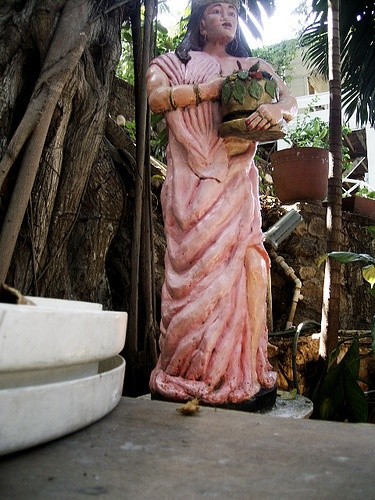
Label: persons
xmin=144 ymin=0 xmax=299 ymax=409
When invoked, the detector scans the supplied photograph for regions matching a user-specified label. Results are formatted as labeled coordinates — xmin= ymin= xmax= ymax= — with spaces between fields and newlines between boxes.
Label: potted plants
xmin=272 ymin=120 xmax=329 ymax=206
